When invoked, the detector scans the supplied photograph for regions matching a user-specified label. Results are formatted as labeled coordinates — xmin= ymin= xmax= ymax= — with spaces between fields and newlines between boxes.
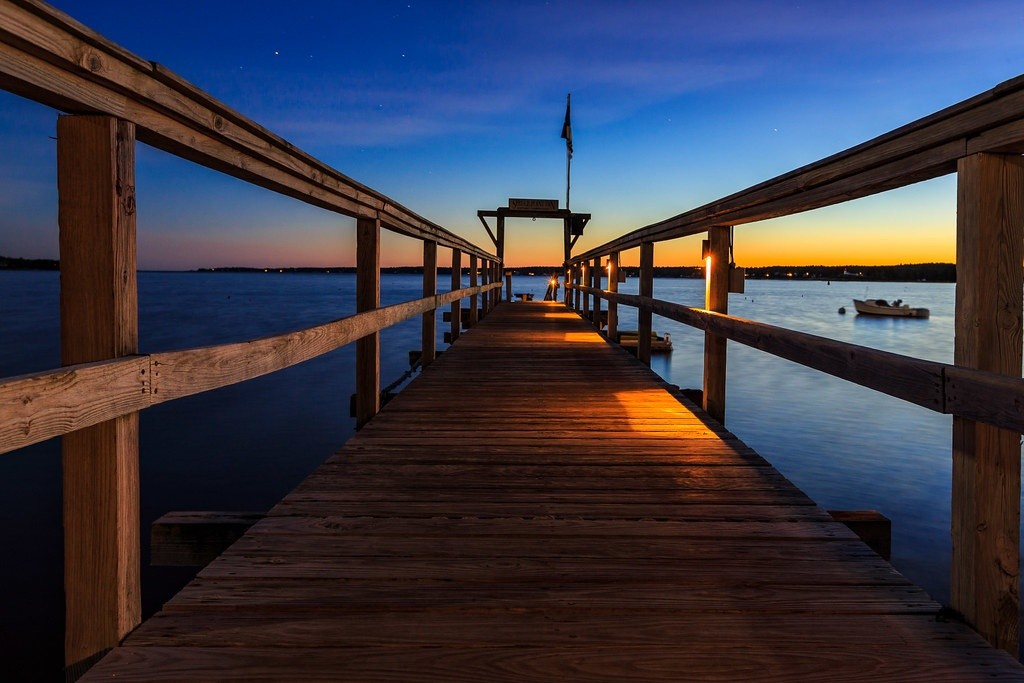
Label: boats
xmin=544 ymin=274 xmax=546 ymax=276
xmin=515 ymin=271 xmax=520 ymax=276
xmin=854 ymin=299 xmax=930 ymax=318
xmin=547 ymin=274 xmax=551 ymax=276
xmin=528 ymin=273 xmax=535 ymax=276
xmin=513 ymin=293 xmax=534 ymax=300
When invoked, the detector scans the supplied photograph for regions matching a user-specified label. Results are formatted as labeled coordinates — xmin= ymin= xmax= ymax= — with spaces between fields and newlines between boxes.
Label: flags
xmin=561 ymin=95 xmax=573 ymax=158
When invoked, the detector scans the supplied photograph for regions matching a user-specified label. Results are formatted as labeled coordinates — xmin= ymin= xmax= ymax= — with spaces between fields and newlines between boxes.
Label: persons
xmin=893 ymin=299 xmax=902 ymax=307
